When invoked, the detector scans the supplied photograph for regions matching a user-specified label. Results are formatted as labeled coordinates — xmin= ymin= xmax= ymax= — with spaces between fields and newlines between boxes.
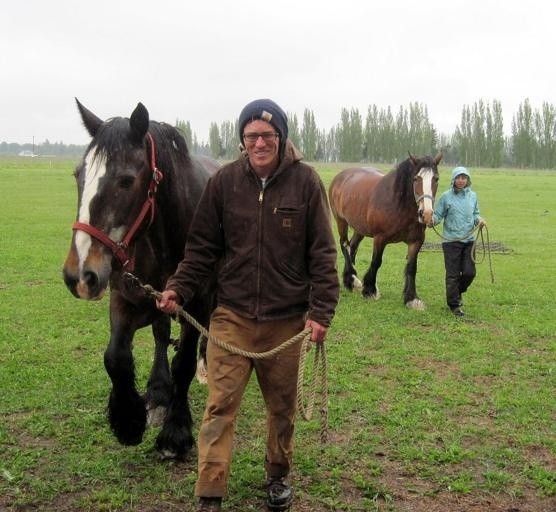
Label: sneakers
xmin=265 ymin=473 xmax=293 ymax=510
xmin=453 ymin=307 xmax=465 ymax=316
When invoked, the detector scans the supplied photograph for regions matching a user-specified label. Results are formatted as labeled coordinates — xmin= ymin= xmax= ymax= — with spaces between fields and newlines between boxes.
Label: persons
xmin=431 ymin=166 xmax=487 ymax=315
xmin=154 ymin=99 xmax=340 ymax=510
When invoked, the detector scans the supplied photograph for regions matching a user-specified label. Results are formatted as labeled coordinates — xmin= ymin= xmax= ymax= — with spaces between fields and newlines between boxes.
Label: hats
xmin=238 ymin=98 xmax=289 ymax=148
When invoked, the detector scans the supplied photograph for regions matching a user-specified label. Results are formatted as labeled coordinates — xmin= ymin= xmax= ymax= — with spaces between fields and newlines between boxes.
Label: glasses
xmin=239 ymin=132 xmax=280 ymax=142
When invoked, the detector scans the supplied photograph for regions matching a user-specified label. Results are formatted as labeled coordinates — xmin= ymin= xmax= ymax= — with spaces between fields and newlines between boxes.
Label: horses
xmin=329 ymin=150 xmax=443 ymax=311
xmin=62 ymin=96 xmax=227 ymax=471
